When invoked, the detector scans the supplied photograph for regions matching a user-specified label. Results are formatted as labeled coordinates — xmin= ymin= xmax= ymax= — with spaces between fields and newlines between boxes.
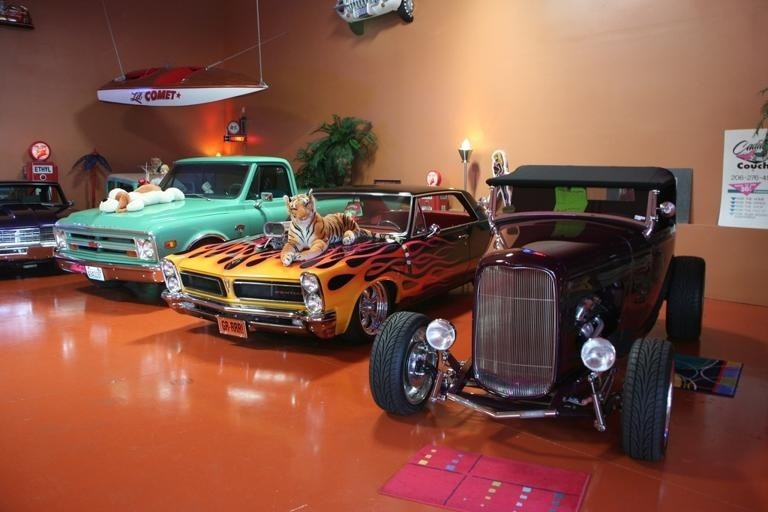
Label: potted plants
xmin=295 ymin=113 xmax=377 ymax=187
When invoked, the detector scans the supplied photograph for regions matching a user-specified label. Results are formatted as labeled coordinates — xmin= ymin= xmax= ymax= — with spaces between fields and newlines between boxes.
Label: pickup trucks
xmin=52 ymin=151 xmax=363 ymax=304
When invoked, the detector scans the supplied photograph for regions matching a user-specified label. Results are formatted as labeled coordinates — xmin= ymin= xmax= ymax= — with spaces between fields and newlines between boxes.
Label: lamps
xmin=458 ymin=138 xmax=474 ymax=192
xmin=224 ymin=135 xmax=246 ymax=142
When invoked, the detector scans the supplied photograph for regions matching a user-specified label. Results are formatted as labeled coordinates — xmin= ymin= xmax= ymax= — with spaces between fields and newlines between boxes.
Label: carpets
xmin=674 ymin=353 xmax=744 ymax=398
xmin=379 ymin=442 xmax=592 ymax=512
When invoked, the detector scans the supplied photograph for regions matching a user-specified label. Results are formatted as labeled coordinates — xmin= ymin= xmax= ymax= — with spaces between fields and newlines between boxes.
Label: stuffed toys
xmin=99 ymin=187 xmax=185 ymax=214
xmin=281 ymin=188 xmax=412 ymax=274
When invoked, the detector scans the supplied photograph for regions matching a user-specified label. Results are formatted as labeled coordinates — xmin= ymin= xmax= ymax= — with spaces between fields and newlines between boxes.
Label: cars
xmin=335 ymin=0 xmax=415 ymax=35
xmin=0 ymin=5 xmax=34 ymax=30
xmin=105 ymin=171 xmax=166 ymax=200
xmin=161 ymin=186 xmax=491 ymax=348
xmin=0 ymin=180 xmax=78 ymax=278
xmin=369 ymin=164 xmax=705 ymax=464
